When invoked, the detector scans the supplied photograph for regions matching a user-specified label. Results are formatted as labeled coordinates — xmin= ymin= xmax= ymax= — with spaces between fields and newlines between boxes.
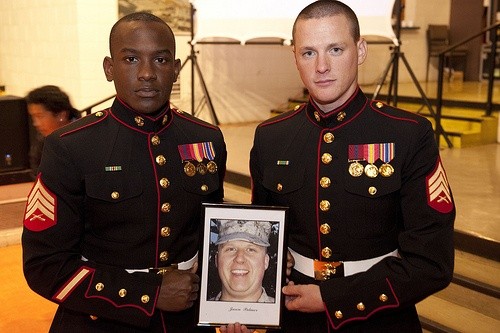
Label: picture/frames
xmin=196 ymin=203 xmax=291 ymax=329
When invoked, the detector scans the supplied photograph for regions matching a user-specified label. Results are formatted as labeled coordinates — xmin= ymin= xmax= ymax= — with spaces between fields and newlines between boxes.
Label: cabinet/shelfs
xmin=0 ymin=95 xmax=39 ymax=185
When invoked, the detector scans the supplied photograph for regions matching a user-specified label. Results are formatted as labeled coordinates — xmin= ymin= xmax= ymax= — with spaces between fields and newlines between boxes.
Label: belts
xmin=81 ymin=251 xmax=198 ymax=274
xmin=288 ymin=246 xmax=399 ymax=281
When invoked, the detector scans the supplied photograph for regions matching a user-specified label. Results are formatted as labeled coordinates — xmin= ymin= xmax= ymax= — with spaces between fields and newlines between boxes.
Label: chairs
xmin=425 ymin=24 xmax=469 ymax=83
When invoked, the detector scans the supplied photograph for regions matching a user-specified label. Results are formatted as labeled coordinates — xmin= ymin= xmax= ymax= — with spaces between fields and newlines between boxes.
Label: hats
xmin=215 ymin=220 xmax=271 ymax=247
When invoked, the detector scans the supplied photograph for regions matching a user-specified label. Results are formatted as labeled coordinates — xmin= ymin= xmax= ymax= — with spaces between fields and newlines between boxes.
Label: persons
xmin=219 ymin=0 xmax=456 ymax=332
xmin=25 ymin=85 xmax=81 ymax=177
xmin=22 ymin=14 xmax=295 ymax=333
xmin=210 ymin=219 xmax=276 ymax=302
